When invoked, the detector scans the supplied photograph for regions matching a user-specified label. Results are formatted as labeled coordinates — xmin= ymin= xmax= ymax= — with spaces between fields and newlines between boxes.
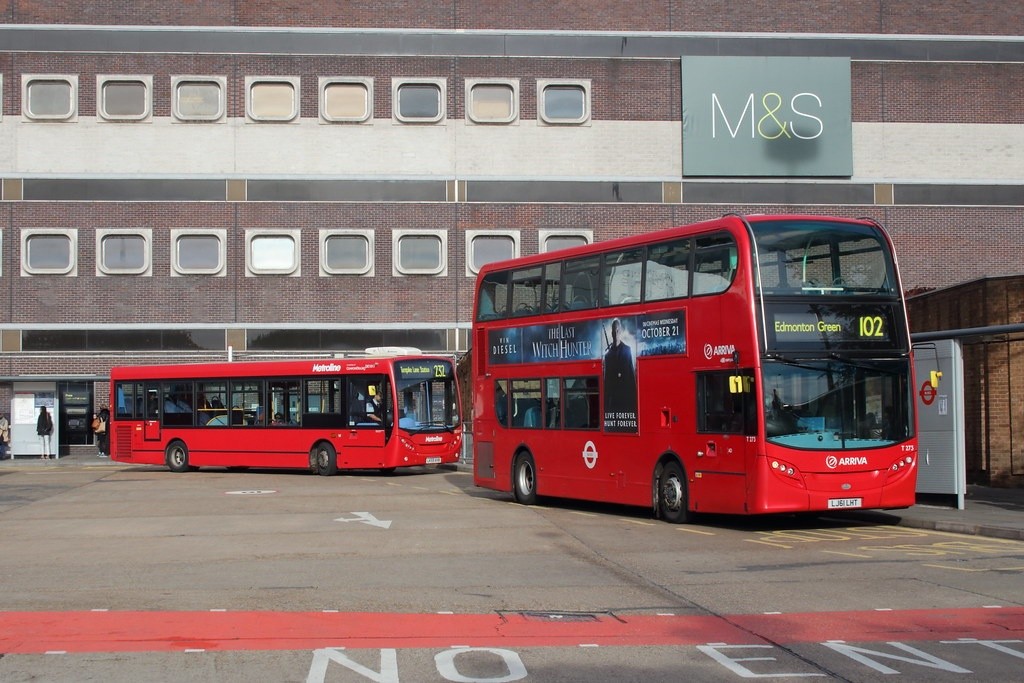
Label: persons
xmin=36 ymin=406 xmax=52 ymax=459
xmin=93 ymin=404 xmax=110 ymax=458
xmin=138 ymin=392 xmax=298 ymax=426
xmin=366 ymin=391 xmax=382 ymax=421
xmin=0 ymin=410 xmax=9 ymax=460
xmin=604 ymin=318 xmax=638 ymax=432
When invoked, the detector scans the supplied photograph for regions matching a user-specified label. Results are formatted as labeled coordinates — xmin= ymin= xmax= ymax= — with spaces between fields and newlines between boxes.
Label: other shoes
xmin=41 ymin=455 xmax=45 ymax=459
xmin=97 ymin=451 xmax=101 ymax=456
xmin=46 ymin=456 xmax=50 ymax=459
xmin=99 ymin=452 xmax=107 ymax=457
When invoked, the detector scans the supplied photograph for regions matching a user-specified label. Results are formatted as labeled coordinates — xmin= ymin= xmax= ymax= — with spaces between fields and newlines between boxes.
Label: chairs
xmin=524 ymin=405 xmax=567 ymax=430
xmin=253 ymin=418 xmax=300 ymax=425
xmin=773 ymin=278 xmax=848 ymax=295
xmin=484 ymin=295 xmax=593 ymax=317
xmin=126 ymin=396 xmax=228 ymax=416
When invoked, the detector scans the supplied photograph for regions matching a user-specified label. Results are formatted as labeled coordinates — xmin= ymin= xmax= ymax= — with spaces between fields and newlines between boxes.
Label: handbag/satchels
xmin=46 ymin=413 xmax=51 ymax=431
xmin=94 ymin=417 xmax=105 ymax=433
xmin=91 ymin=417 xmax=100 ymax=430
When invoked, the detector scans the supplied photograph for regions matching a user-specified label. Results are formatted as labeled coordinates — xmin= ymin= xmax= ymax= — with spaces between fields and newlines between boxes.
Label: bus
xmin=110 ymin=361 xmax=465 ymax=476
xmin=468 ymin=211 xmax=921 ymax=525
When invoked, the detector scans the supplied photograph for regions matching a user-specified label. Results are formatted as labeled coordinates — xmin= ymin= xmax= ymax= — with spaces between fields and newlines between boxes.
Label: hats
xmin=100 ymin=404 xmax=107 ymax=409
